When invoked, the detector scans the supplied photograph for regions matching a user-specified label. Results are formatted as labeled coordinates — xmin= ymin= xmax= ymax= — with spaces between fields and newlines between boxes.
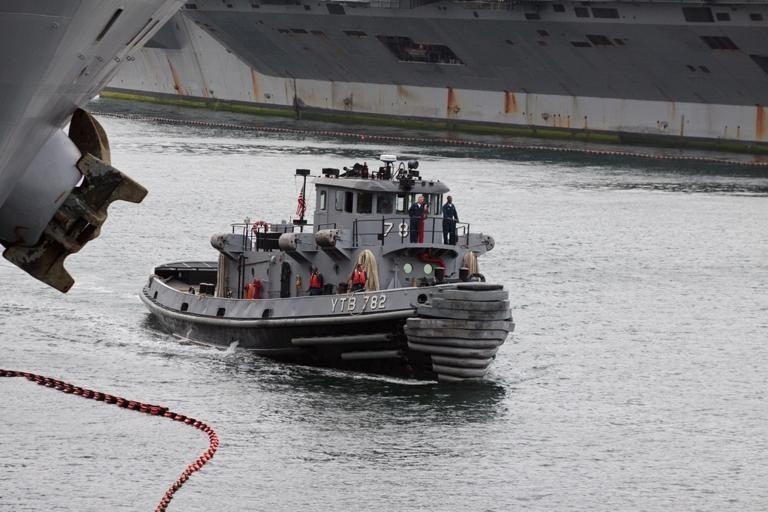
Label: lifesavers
xmin=253 ymin=220 xmax=268 ymax=235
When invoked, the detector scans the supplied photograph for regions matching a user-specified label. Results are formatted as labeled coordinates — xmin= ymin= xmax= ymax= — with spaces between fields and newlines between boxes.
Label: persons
xmin=408 ymin=195 xmax=426 ymax=242
xmin=350 ymin=263 xmax=368 ymax=291
xmin=441 ymin=196 xmax=460 ymax=246
xmin=305 ymin=265 xmax=324 ymax=295
xmin=418 ymin=201 xmax=430 ymax=242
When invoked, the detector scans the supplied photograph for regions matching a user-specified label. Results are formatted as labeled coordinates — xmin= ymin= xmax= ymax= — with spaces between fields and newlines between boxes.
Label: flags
xmin=295 ymin=184 xmax=306 ymax=218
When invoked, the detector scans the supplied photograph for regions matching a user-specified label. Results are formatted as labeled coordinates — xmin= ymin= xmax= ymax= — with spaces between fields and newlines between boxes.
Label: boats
xmin=139 ymin=155 xmax=514 ymax=383
